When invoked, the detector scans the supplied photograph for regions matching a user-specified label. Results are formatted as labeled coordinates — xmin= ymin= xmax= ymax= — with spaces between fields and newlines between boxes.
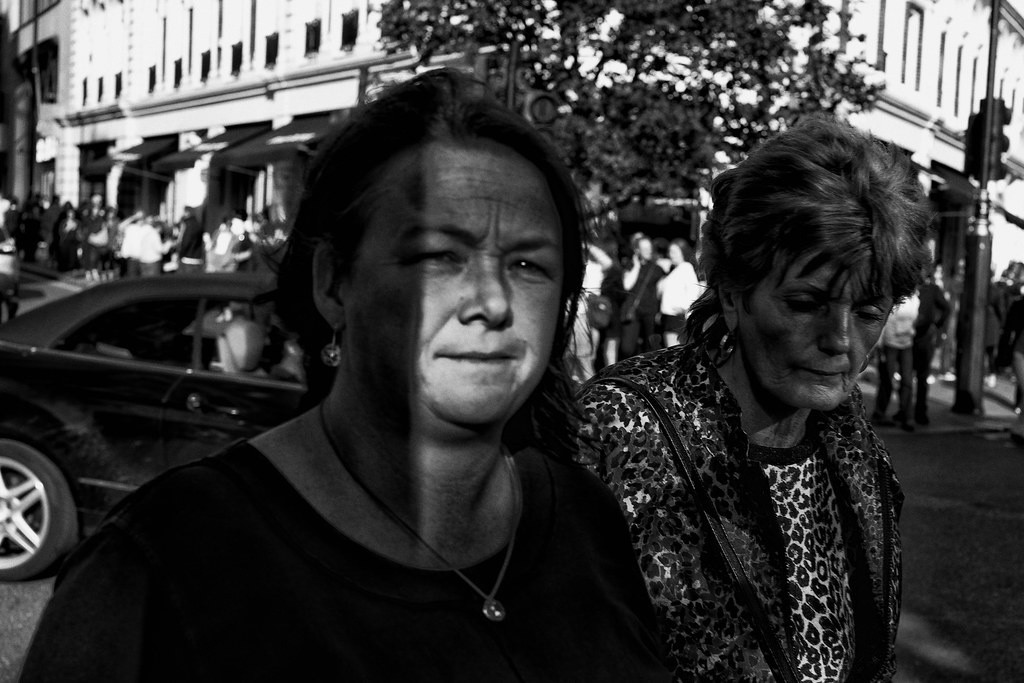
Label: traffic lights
xmin=978 ymin=98 xmax=1011 ymax=181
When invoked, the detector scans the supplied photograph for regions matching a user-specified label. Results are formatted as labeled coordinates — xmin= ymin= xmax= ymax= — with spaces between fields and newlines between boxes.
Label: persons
xmin=982 ymin=283 xmax=1024 ymax=415
xmin=564 ymin=119 xmax=933 ymax=683
xmin=869 ymin=283 xmax=951 ymax=432
xmin=19 ymin=65 xmax=681 ymax=683
xmin=565 ymin=231 xmax=700 ymax=383
xmin=0 ymin=191 xmax=274 ymax=321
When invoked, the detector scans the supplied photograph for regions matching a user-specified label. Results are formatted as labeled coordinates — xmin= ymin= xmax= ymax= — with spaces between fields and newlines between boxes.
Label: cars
xmin=0 ymin=193 xmax=24 ymax=328
xmin=0 ymin=266 xmax=587 ymax=586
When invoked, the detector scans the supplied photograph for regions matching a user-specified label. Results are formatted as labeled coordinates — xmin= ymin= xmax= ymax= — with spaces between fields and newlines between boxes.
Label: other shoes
xmin=900 ymin=420 xmax=914 ymax=432
xmin=915 ymin=414 xmax=929 ymax=425
xmin=893 ymin=411 xmax=903 ymax=420
xmin=869 ymin=412 xmax=885 ymax=424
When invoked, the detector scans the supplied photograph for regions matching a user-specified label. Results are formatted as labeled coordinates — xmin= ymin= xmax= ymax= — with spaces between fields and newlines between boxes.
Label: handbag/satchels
xmin=612 ymin=291 xmax=641 ymax=324
xmin=921 ymin=296 xmax=950 ymax=347
xmin=587 ymin=293 xmax=612 ymax=328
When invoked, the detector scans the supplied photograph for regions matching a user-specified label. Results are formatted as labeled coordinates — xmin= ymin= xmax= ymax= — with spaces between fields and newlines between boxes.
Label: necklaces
xmin=320 ymin=401 xmax=520 ymax=621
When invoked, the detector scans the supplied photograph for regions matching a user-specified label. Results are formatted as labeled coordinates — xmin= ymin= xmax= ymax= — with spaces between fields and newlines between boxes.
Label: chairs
xmin=218 ymin=316 xmax=266 ymax=377
